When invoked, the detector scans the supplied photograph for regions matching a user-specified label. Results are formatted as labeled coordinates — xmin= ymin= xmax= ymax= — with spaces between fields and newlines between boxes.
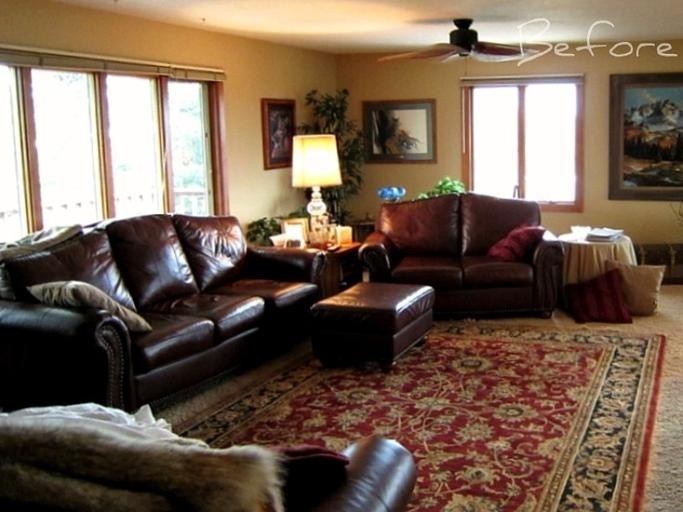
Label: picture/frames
xmin=361 ymin=98 xmax=437 ymax=164
xmin=260 ymin=98 xmax=295 ymax=170
xmin=609 ymin=72 xmax=682 ymax=201
xmin=279 ymin=217 xmax=308 ymax=247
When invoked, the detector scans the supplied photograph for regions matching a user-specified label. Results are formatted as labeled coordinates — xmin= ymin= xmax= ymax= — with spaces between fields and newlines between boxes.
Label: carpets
xmin=169 ymin=323 xmax=665 ymax=509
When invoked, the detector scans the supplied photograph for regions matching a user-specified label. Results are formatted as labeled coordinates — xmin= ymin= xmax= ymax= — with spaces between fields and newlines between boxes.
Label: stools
xmin=310 ymin=281 xmax=433 ymax=371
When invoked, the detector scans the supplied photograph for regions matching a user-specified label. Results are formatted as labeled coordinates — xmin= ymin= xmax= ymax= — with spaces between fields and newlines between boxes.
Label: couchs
xmin=0 ymin=211 xmax=329 ymax=411
xmin=0 ymin=400 xmax=415 ymax=509
xmin=357 ymin=193 xmax=564 ymax=320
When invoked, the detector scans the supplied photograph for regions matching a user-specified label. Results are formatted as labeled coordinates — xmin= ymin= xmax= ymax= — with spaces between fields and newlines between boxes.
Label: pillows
xmin=259 ymin=439 xmax=348 ymax=492
xmin=23 ymin=281 xmax=152 ymax=331
xmin=566 ymin=270 xmax=633 ymax=323
xmin=604 ymin=260 xmax=666 ymax=316
xmin=487 ymin=222 xmax=544 ymax=259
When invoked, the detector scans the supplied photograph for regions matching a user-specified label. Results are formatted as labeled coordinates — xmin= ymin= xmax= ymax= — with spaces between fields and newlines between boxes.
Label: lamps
xmin=290 ymin=134 xmax=342 ymax=231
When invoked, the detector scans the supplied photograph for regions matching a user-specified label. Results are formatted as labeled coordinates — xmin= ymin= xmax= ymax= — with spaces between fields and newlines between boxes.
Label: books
xmin=585 ymin=227 xmax=623 ymax=242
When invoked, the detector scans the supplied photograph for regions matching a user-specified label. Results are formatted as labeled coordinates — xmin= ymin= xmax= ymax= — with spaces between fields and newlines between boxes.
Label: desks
xmin=560 ymin=235 xmax=638 ymax=284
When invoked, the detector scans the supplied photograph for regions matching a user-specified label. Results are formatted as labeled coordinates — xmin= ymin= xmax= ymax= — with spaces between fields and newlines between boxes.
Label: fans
xmin=377 ymin=16 xmax=533 ymax=64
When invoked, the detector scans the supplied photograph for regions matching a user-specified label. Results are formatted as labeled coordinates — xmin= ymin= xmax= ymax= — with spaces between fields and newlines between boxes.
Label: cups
xmin=337 ymin=226 xmax=351 ymax=246
xmin=308 ymin=232 xmax=328 ymax=251
